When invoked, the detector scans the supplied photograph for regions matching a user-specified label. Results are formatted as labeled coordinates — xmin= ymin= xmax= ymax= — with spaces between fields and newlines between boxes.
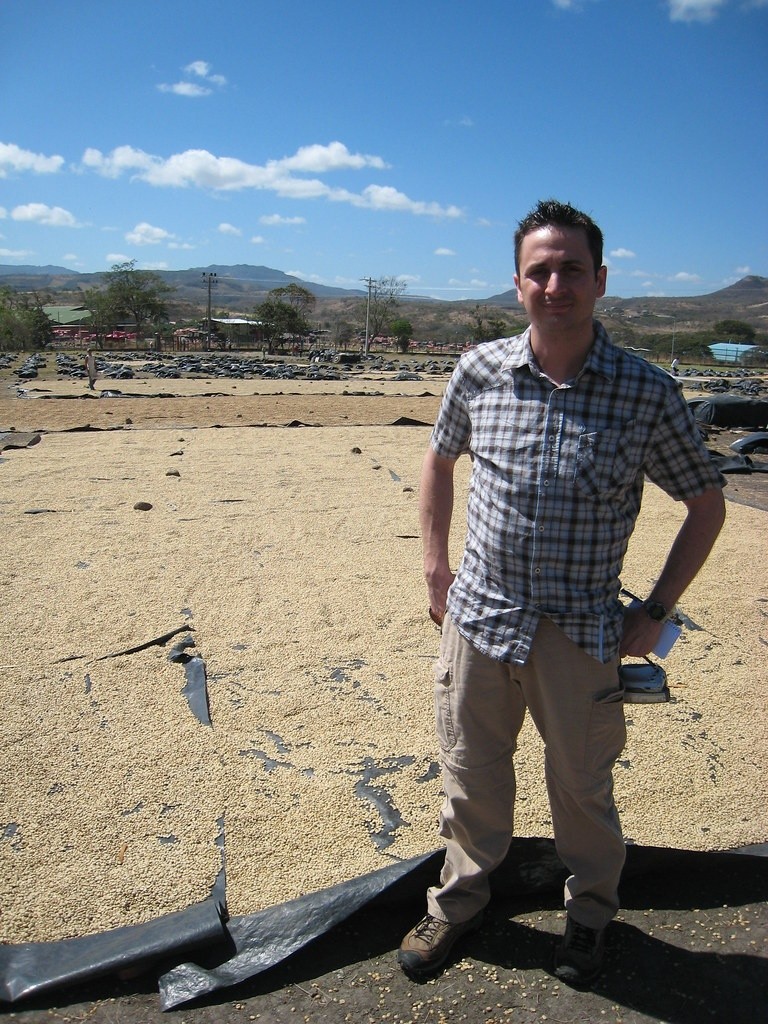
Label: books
xmin=628 ymin=600 xmax=681 ymax=660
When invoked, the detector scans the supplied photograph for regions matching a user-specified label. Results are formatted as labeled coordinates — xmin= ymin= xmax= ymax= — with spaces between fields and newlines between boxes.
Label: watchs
xmin=645 ymin=599 xmax=668 ymax=624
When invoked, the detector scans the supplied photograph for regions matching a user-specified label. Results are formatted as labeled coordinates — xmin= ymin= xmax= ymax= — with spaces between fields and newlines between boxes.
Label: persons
xmin=399 ymin=200 xmax=728 ymax=984
xmin=671 ymin=357 xmax=680 ymax=374
xmin=86 ymin=348 xmax=98 ymax=389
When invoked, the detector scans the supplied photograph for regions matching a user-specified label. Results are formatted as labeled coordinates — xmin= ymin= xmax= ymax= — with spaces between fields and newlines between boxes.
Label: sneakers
xmin=554 ymin=915 xmax=606 ymax=981
xmin=398 ymin=910 xmax=478 ymax=971
